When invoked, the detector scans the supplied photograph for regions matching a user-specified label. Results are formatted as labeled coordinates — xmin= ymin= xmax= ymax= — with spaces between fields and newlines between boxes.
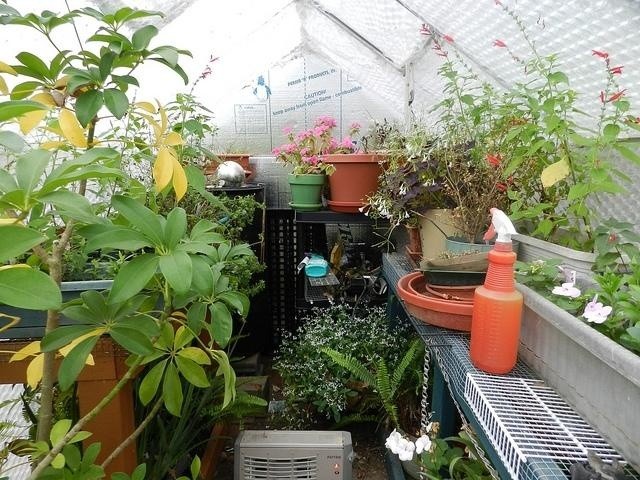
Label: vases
xmin=207 ymin=152 xmax=254 ymax=182
xmin=515 ymin=267 xmax=640 ymax=475
xmin=0 ymin=280 xmax=116 ymax=339
xmin=319 ymin=151 xmax=392 ymax=213
xmin=287 ymin=173 xmax=324 ymax=212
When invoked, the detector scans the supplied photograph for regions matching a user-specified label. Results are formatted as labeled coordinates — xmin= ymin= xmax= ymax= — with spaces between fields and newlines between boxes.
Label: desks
xmin=0 ymin=310 xmax=223 ymax=480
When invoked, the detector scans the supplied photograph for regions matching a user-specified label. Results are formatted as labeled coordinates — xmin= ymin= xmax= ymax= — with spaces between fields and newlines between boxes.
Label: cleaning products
xmin=303 ymin=251 xmax=330 ymax=278
xmin=469 ymin=207 xmax=524 ymax=374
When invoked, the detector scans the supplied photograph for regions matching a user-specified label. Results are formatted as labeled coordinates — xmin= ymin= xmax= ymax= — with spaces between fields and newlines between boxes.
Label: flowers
xmin=271 ymin=115 xmax=368 ymax=178
xmin=265 ymin=290 xmax=418 ymax=430
xmin=513 ymin=257 xmax=640 ymax=355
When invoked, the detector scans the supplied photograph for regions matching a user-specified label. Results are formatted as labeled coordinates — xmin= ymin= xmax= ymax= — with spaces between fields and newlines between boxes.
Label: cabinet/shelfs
xmin=382 ymin=250 xmax=640 ymax=480
xmin=236 ymin=208 xmax=404 ymax=355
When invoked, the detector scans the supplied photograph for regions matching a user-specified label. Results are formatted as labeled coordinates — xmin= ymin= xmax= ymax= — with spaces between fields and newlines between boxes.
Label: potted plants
xmin=357 ymin=0 xmax=640 ymax=279
